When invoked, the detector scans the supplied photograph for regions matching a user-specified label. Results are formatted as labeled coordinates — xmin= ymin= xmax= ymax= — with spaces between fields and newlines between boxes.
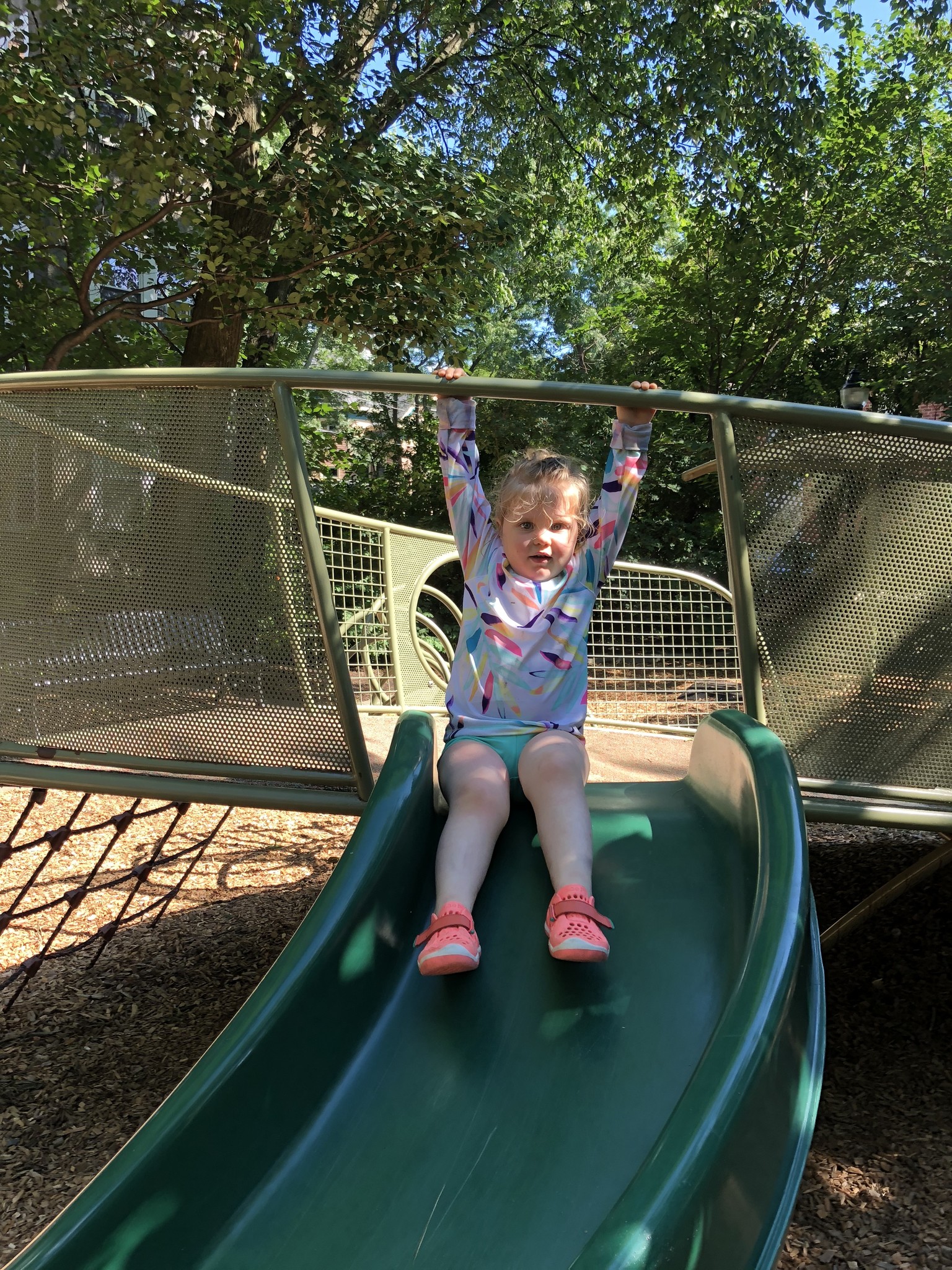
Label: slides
xmin=0 ymin=710 xmax=829 ymax=1269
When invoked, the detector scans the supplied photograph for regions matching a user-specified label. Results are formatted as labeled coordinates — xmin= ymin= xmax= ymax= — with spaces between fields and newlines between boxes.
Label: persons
xmin=411 ymin=364 xmax=664 ymax=977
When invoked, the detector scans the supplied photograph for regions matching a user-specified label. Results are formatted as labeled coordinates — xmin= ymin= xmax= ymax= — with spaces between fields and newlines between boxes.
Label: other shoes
xmin=414 ymin=902 xmax=482 ymax=976
xmin=544 ymin=885 xmax=615 ymax=961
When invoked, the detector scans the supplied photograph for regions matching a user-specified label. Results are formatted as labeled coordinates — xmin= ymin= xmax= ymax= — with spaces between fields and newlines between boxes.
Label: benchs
xmin=0 ymin=608 xmax=272 ymax=737
